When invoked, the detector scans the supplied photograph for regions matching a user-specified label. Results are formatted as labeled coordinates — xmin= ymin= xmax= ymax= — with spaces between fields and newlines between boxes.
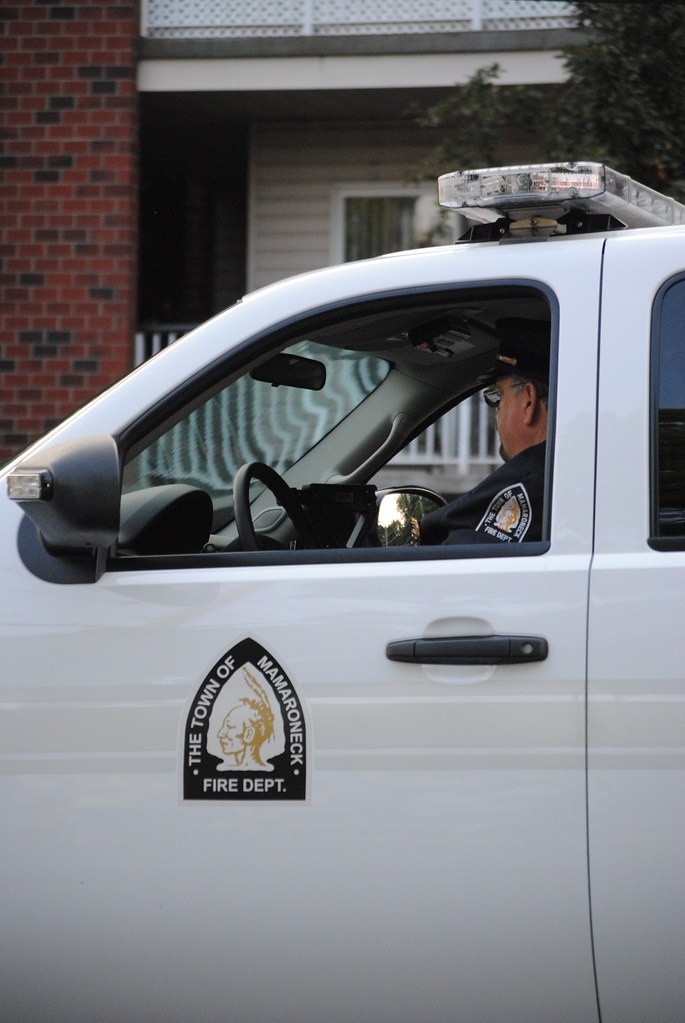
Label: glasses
xmin=481 ymin=381 xmax=527 ymax=408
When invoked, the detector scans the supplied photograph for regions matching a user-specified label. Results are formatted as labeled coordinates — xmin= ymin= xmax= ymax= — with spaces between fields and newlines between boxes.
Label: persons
xmin=302 ymin=317 xmax=550 ymax=548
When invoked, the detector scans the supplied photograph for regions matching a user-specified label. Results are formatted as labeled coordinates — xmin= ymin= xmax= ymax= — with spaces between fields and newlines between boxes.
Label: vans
xmin=1 ymin=161 xmax=685 ymax=1022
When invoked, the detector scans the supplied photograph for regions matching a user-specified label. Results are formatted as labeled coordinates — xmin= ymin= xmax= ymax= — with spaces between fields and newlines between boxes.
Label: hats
xmin=476 ymin=317 xmax=551 ymax=382
